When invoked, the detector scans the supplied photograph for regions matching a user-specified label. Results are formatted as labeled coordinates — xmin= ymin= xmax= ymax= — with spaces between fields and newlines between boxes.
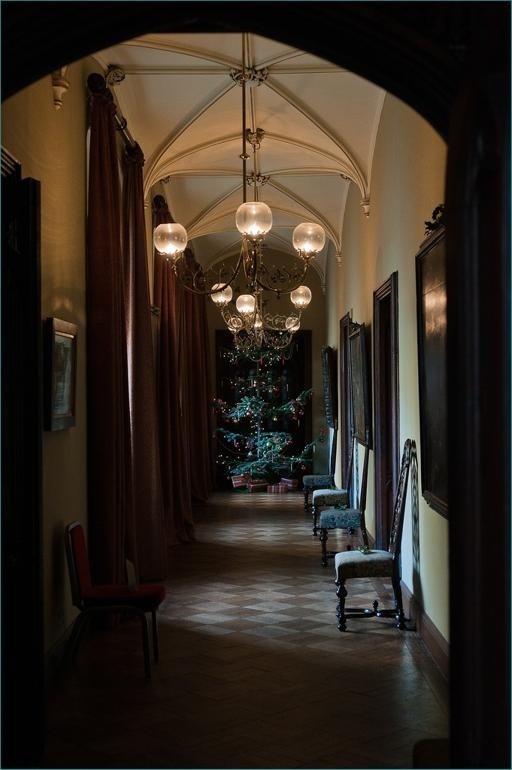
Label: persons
xmin=52 ymin=342 xmax=66 ymax=395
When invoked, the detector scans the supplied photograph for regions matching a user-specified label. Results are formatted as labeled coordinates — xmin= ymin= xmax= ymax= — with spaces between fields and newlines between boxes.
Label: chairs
xmin=303 ymin=418 xmax=412 ymax=632
xmin=64 ymin=520 xmax=167 ymax=680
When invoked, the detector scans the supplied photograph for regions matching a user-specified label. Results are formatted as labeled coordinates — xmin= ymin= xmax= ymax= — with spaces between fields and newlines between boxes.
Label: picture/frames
xmin=347 ymin=322 xmax=372 ymax=450
xmin=41 ymin=316 xmax=79 ymax=434
xmin=415 ymin=220 xmax=448 ymax=519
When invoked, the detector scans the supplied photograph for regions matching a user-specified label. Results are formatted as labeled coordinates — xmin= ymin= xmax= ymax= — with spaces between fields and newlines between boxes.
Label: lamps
xmin=153 ymin=33 xmax=326 ymax=365
